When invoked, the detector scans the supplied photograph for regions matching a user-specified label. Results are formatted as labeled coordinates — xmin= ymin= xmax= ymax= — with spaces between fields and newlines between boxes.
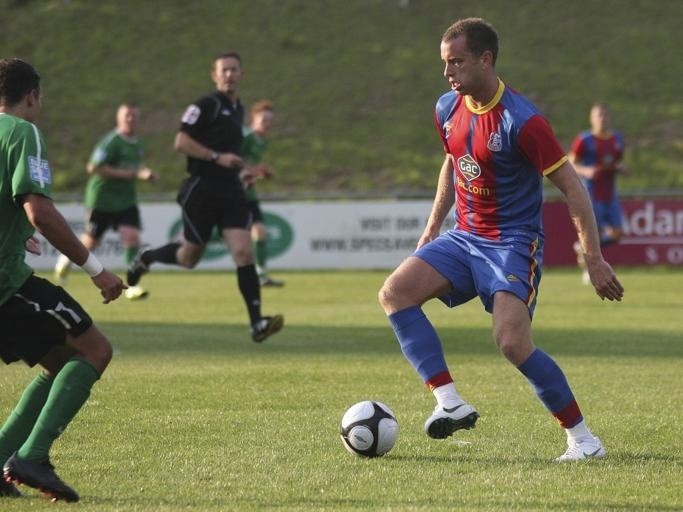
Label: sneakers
xmin=56 ymin=253 xmax=71 ymax=278
xmin=425 ymin=402 xmax=480 ymax=438
xmin=260 ymin=277 xmax=283 ymax=286
xmin=251 ymin=315 xmax=283 ymax=342
xmin=556 ymin=436 xmax=605 ymax=460
xmin=125 ymin=244 xmax=152 ymax=299
xmin=0 ymin=450 xmax=78 ymax=501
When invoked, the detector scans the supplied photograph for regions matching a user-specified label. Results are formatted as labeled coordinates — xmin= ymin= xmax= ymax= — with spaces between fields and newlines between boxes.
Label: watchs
xmin=209 ymin=149 xmax=222 ymax=165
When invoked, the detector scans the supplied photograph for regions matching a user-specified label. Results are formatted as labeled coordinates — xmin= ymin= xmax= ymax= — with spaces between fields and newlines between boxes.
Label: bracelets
xmin=76 ymin=249 xmax=105 ymax=280
xmin=128 ymin=168 xmax=138 ymax=181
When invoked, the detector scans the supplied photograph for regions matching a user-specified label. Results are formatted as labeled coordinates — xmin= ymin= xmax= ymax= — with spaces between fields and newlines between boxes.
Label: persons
xmin=377 ymin=18 xmax=625 ymax=462
xmin=209 ymin=98 xmax=286 ymax=289
xmin=125 ymin=52 xmax=283 ymax=344
xmin=563 ymin=104 xmax=628 ymax=286
xmin=0 ymin=56 xmax=130 ymax=505
xmin=53 ymin=101 xmax=163 ymax=300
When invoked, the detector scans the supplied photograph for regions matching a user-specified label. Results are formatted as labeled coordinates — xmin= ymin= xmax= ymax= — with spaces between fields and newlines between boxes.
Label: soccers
xmin=340 ymin=400 xmax=400 ymax=458
